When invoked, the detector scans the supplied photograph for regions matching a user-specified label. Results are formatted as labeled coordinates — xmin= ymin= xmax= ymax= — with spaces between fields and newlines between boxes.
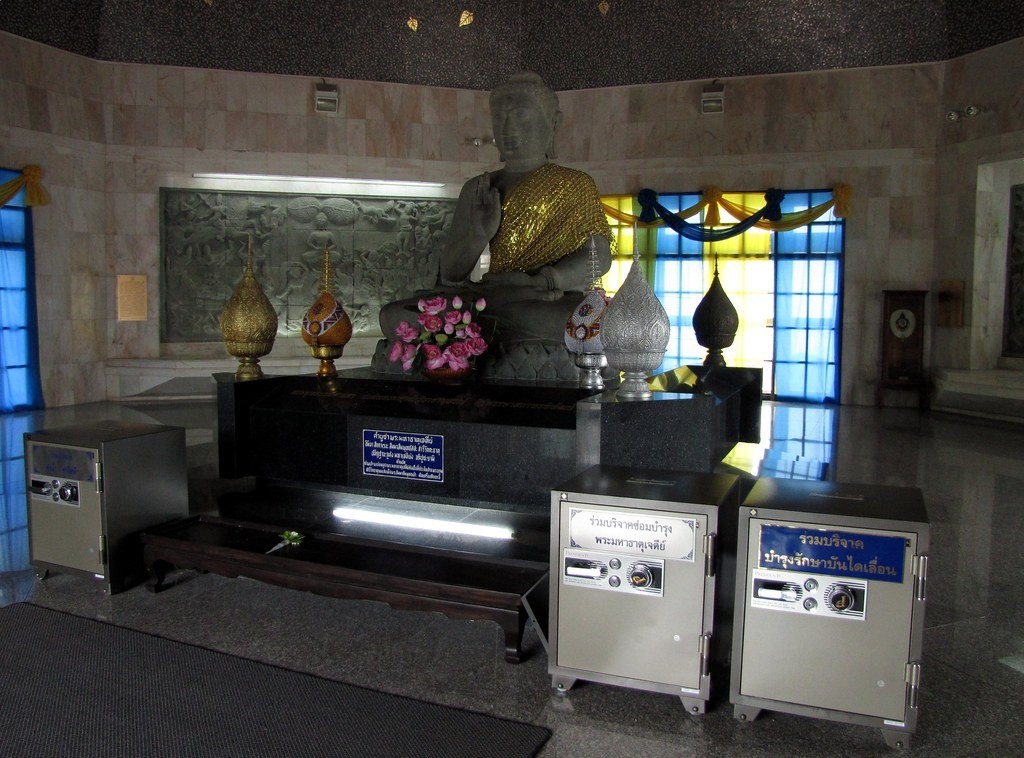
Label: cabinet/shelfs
xmin=600 ymin=365 xmax=763 ymax=473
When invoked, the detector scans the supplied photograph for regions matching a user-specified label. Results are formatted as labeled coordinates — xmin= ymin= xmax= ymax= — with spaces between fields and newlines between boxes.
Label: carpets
xmin=0 ymin=601 xmax=552 ymax=758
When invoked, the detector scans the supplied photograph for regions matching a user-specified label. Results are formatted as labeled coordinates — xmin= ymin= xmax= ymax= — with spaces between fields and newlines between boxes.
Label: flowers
xmin=266 ymin=530 xmax=306 ymax=555
xmin=390 ymin=296 xmax=498 ymax=373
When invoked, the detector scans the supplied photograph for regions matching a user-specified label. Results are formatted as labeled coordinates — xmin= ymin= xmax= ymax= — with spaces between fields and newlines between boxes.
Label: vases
xmin=421 ymin=369 xmax=475 ymax=387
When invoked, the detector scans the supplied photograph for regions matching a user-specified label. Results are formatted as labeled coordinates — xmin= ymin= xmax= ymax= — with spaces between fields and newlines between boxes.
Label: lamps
xmin=472 ymin=139 xmax=484 ymax=148
xmin=491 ymin=138 xmax=498 ymax=147
xmin=314 ymin=82 xmax=339 ymax=113
xmin=700 ymin=82 xmax=725 ymax=113
xmin=946 ymin=110 xmax=960 ymax=124
xmin=965 ymin=104 xmax=979 ymax=118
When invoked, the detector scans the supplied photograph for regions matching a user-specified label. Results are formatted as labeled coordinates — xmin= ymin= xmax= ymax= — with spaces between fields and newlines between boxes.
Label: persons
xmin=379 ymin=70 xmax=612 ymax=345
